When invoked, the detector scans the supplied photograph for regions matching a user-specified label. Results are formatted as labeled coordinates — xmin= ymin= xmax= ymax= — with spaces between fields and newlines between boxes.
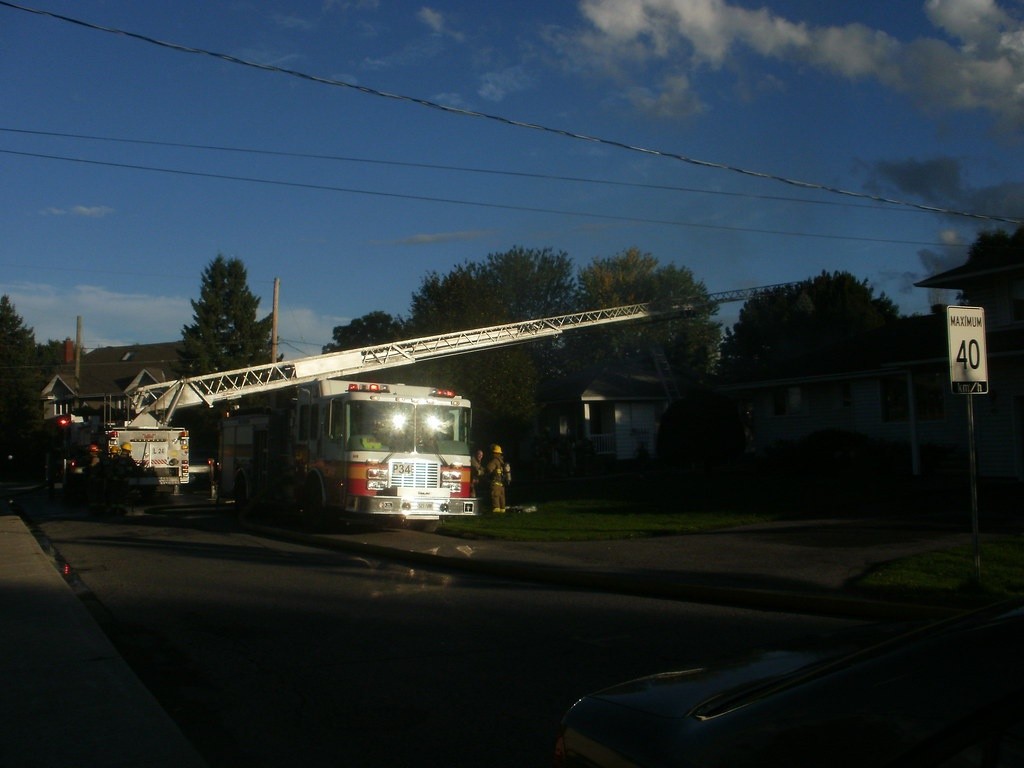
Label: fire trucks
xmin=214 ymin=375 xmax=484 ymax=527
xmin=36 ymin=272 xmax=847 ymax=516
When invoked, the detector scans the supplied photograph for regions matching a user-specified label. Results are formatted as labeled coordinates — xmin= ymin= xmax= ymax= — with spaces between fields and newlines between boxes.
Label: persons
xmin=485 ymin=444 xmax=509 ymax=513
xmin=84 ymin=442 xmax=138 ymax=518
xmin=472 ymin=450 xmax=487 ymax=510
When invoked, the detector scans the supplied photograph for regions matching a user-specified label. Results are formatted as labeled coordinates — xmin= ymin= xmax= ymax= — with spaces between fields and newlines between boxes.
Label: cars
xmin=554 ymin=593 xmax=1022 ymax=768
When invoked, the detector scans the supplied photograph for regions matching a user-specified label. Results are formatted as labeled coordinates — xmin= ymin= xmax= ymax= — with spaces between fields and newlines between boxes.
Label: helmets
xmin=490 ymin=443 xmax=502 ymax=454
